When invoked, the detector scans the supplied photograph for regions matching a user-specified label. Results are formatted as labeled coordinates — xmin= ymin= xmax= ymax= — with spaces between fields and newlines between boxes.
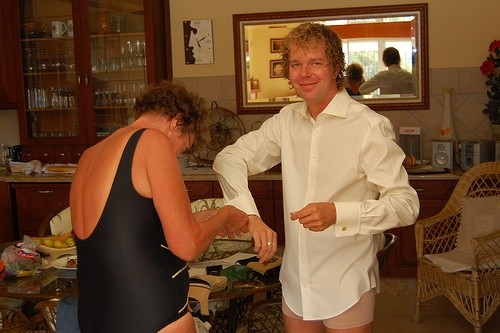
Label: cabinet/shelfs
xmin=13 ymin=0 xmax=167 ymax=165
xmin=0 ymin=179 xmax=500 ymax=279
xmin=0 ymin=0 xmax=17 ymax=110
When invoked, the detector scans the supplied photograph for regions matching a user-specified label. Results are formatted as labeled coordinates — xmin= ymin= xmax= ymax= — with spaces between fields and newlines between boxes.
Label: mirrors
xmin=232 ymin=4 xmax=430 ymax=115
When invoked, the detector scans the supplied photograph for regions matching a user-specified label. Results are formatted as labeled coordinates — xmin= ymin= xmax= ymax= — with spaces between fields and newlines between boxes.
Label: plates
xmin=404 ymin=159 xmax=430 ymax=169
xmin=30 ymin=237 xmax=76 ymax=252
xmin=51 ymin=255 xmax=77 ymax=270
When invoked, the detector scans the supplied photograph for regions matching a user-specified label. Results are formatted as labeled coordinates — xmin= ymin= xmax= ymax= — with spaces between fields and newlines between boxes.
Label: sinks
xmin=266 ymin=163 xmax=281 ymax=174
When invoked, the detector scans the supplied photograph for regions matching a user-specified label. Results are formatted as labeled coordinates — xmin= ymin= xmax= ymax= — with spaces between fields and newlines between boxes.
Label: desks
xmin=0 ymin=239 xmax=284 ymax=333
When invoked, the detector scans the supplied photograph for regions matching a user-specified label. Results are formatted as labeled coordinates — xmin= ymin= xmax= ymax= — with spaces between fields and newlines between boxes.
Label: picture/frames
xmin=270 ymin=60 xmax=285 ymax=78
xmin=270 ymin=38 xmax=283 ymax=53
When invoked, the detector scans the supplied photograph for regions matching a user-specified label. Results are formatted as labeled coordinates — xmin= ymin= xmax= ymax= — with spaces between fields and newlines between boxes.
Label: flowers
xmin=480 ymin=40 xmax=500 ymax=124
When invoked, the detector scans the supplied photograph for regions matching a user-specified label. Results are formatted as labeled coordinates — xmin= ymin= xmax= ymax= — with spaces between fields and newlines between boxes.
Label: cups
xmin=22 ymin=19 xmax=74 ymax=39
xmin=97 ymin=12 xmax=129 ymax=34
xmin=31 ymin=122 xmax=80 ymax=137
xmin=28 ymin=86 xmax=76 ymax=108
xmin=91 ymin=39 xmax=146 ymax=72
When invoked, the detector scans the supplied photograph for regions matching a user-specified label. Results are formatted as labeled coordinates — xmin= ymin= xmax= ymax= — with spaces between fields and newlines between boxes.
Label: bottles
xmin=438 ymin=86 xmax=459 ymax=166
xmin=12 ymin=144 xmax=21 ymax=162
xmin=31 ymin=114 xmax=41 ymax=137
xmin=0 ymin=143 xmax=8 ymax=171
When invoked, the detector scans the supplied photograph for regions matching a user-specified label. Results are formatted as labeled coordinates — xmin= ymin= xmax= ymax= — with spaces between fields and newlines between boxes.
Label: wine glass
xmin=22 ymin=45 xmax=75 ymax=71
xmin=93 ymin=83 xmax=146 ymax=107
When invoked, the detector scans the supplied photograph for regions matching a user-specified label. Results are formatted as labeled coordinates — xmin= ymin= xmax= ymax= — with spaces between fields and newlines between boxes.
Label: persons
xmin=70 ymin=79 xmax=250 ymax=333
xmin=359 ymin=47 xmax=413 ymax=95
xmin=339 ymin=62 xmax=372 ymax=96
xmin=210 ymin=21 xmax=420 ymax=333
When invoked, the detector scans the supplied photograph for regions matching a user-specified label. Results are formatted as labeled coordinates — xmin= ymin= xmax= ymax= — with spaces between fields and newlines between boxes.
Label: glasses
xmin=180 ymin=130 xmax=194 ymax=155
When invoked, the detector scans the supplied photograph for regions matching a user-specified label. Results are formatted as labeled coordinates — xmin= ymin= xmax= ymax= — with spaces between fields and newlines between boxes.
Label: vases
xmin=491 ymin=125 xmax=500 ymax=135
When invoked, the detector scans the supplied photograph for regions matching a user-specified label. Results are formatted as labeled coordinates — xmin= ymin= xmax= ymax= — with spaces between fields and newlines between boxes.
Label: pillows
xmin=455 ymin=195 xmax=500 ymax=255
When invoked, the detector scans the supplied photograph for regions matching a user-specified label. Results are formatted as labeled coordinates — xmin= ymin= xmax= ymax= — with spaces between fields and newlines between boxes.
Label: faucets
xmin=251 ymin=121 xmax=262 ymax=131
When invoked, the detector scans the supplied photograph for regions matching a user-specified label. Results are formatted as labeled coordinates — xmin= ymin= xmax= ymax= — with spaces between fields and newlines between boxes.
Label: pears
xmin=34 ymin=238 xmax=76 ymax=249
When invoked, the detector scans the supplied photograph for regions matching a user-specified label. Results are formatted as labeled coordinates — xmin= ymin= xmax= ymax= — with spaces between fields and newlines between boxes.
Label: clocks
xmin=183 ymin=18 xmax=214 ymax=65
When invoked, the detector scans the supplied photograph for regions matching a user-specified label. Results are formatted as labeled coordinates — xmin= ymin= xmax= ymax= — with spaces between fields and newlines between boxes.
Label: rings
xmin=267 ymin=241 xmax=273 ymax=246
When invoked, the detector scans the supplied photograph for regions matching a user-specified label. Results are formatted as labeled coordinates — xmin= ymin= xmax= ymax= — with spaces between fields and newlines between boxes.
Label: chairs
xmin=233 ymin=233 xmax=400 ymax=333
xmin=415 ymin=162 xmax=500 ymax=333
